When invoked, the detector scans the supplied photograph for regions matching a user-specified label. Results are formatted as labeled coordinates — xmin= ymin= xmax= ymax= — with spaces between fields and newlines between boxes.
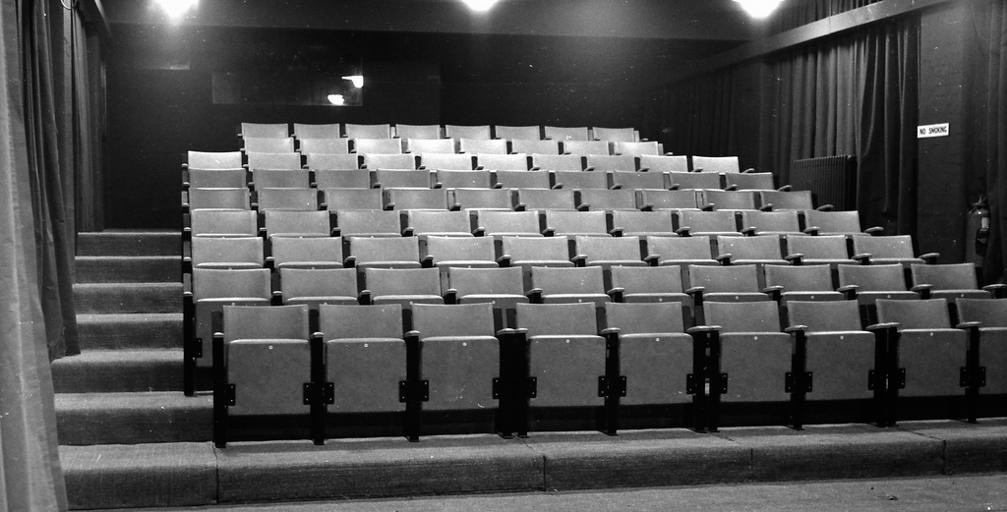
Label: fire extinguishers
xmin=951 ymin=191 xmax=993 ymax=268
